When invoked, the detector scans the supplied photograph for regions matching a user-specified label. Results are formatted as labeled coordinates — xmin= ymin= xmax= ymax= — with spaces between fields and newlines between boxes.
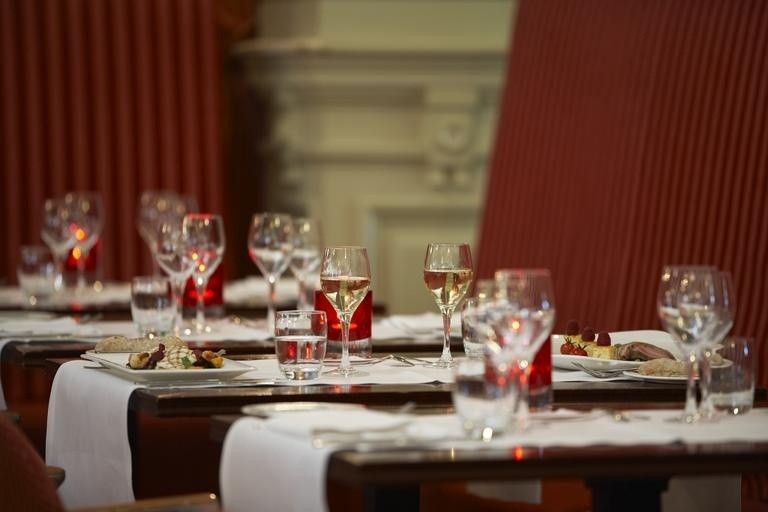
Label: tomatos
xmin=572 ymin=344 xmax=588 ymax=356
xmin=560 ymin=336 xmax=575 ymax=354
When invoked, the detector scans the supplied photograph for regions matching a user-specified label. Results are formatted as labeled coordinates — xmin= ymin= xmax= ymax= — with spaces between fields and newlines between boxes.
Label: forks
xmin=326 ymin=355 xmax=412 ymax=368
xmin=572 ymin=362 xmax=638 ymax=380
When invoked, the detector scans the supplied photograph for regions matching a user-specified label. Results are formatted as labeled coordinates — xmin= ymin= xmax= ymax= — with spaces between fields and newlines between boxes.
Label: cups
xmin=707 ymin=337 xmax=757 ymax=419
xmin=321 ymin=247 xmax=372 ymax=372
xmin=658 ymin=264 xmax=735 ymax=425
xmin=248 ymin=213 xmax=293 ymax=336
xmin=275 ymin=312 xmax=327 ymax=380
xmin=129 ymin=277 xmax=171 ymax=336
xmin=18 ymin=244 xmax=58 ymax=309
xmin=44 ymin=190 xmax=101 ymax=303
xmin=462 ymin=267 xmax=554 ymax=439
xmin=288 ymin=214 xmax=324 ymax=312
xmin=423 ymin=244 xmax=474 ymax=367
xmin=141 ymin=192 xmax=222 ymax=342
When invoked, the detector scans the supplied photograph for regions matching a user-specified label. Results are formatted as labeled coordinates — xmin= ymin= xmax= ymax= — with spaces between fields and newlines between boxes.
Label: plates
xmin=80 ymin=349 xmax=255 ymax=381
xmin=620 ymin=371 xmax=700 ymax=385
xmin=242 ymin=402 xmax=366 ymax=420
xmin=554 ymin=329 xmax=734 ymax=371
